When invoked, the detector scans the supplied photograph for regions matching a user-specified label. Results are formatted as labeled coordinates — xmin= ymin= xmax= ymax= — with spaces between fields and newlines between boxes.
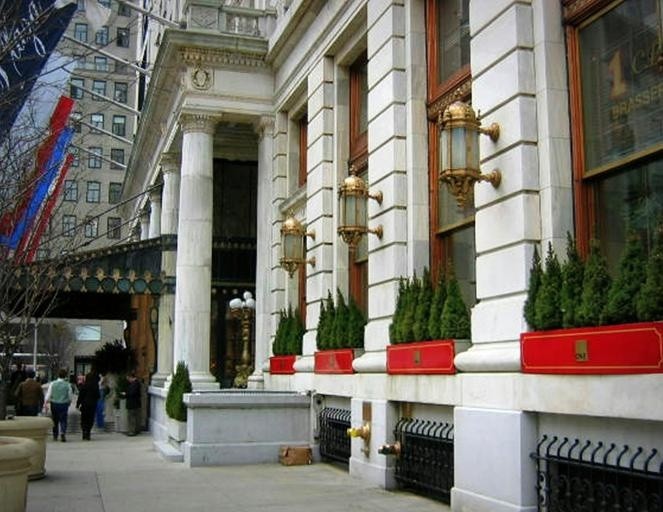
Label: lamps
xmin=276 ymin=207 xmax=318 ymax=278
xmin=437 ymin=98 xmax=503 ymax=210
xmin=333 ymin=162 xmax=385 ymax=254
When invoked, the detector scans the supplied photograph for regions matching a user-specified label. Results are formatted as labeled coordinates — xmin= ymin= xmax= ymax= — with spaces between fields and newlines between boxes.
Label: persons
xmin=11 ymin=363 xmax=106 ymax=442
xmin=116 ymin=371 xmax=142 ymax=436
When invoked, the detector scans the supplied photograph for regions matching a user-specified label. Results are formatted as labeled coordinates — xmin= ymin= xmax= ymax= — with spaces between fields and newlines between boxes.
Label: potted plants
xmin=164 ymin=359 xmax=193 ymax=443
xmin=265 ymin=286 xmax=367 ymax=374
xmin=518 ymin=201 xmax=663 ymax=373
xmin=387 ymin=260 xmax=473 ymax=375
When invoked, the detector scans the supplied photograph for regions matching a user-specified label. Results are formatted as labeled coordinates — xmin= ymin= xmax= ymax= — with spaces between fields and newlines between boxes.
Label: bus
xmin=0 ymin=352 xmax=60 ymax=382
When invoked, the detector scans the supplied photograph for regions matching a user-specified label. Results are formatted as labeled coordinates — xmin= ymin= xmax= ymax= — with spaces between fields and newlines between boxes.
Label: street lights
xmin=229 ymin=291 xmax=256 ymax=388
